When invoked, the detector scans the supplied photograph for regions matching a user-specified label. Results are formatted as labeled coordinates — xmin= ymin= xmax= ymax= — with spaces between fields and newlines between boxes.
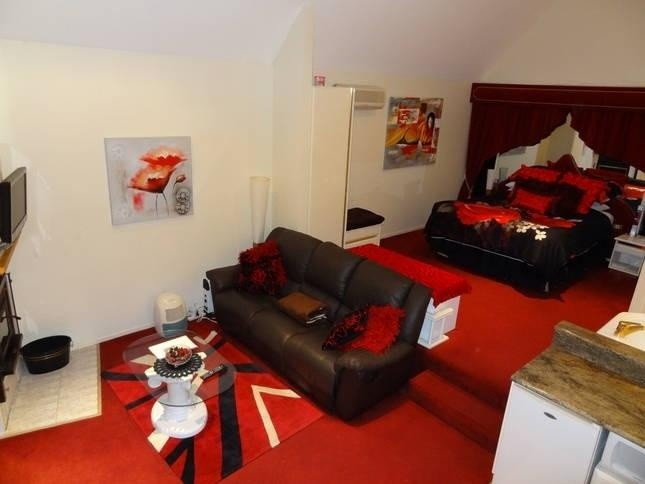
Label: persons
xmin=418 ymin=112 xmax=437 ymax=150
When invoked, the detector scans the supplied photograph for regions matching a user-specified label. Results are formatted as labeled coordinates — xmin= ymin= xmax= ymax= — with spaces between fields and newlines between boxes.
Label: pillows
xmin=505 ymin=164 xmax=611 ymax=216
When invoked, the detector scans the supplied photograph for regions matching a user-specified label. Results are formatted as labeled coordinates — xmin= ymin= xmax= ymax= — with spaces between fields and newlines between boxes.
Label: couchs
xmin=206 ymin=226 xmax=431 ymax=424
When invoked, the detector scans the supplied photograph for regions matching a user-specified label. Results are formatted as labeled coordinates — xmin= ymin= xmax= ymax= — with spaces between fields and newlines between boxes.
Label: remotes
xmin=201 ymin=364 xmax=224 ymax=381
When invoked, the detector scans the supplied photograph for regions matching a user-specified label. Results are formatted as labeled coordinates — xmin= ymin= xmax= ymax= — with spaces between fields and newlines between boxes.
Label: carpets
xmin=101 ymin=329 xmax=324 ymax=484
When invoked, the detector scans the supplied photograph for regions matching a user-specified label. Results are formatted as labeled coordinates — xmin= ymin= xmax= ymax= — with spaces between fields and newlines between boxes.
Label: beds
xmin=422 ymin=153 xmax=617 ymax=287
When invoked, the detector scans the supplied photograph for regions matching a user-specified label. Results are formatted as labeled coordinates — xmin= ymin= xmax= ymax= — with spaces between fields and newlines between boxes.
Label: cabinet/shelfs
xmin=0 ymin=242 xmax=25 ymax=404
xmin=490 ymin=311 xmax=645 ymax=484
xmin=344 ymin=206 xmax=386 ymax=250
xmin=610 ymin=230 xmax=645 ymax=276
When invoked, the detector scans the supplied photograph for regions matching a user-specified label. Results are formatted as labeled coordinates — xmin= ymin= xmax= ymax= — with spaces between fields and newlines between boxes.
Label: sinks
xmin=597 ymin=312 xmax=645 ymax=352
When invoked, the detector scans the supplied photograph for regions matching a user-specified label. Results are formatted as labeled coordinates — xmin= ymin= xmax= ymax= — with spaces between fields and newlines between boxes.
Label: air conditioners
xmin=332 ymin=83 xmax=385 ymax=112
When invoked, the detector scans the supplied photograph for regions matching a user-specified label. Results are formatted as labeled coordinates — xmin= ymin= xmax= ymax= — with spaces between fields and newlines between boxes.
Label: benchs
xmin=349 ymin=244 xmax=470 ymax=349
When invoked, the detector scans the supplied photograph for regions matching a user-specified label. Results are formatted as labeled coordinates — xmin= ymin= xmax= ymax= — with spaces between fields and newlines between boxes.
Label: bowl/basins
xmin=19 ymin=335 xmax=72 ymax=376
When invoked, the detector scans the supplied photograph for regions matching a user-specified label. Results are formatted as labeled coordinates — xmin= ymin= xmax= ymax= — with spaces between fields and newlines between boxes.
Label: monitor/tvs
xmin=0 ymin=168 xmax=28 ymax=248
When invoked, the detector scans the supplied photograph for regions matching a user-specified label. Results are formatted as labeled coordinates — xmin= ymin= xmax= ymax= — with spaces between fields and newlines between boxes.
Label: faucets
xmin=614 ymin=321 xmax=645 ymax=340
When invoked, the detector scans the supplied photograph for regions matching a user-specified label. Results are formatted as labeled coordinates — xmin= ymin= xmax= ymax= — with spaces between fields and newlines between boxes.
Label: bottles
xmin=630 ymin=223 xmax=638 ymax=238
